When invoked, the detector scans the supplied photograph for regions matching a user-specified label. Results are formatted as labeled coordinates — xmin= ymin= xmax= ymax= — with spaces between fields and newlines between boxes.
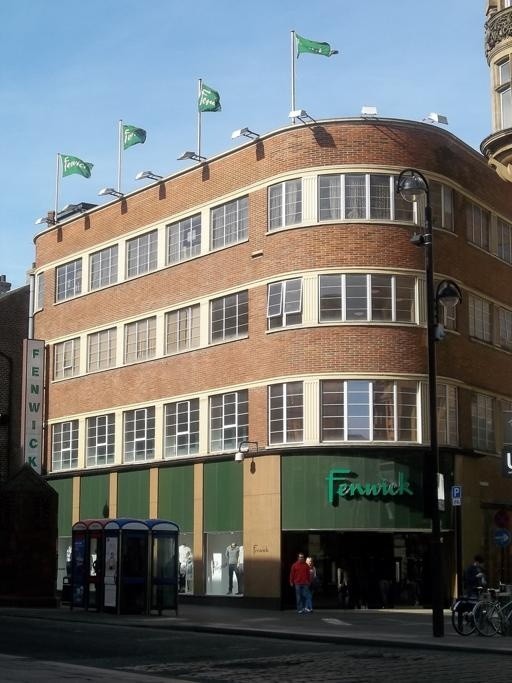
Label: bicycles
xmin=450 ymin=585 xmax=512 ymax=637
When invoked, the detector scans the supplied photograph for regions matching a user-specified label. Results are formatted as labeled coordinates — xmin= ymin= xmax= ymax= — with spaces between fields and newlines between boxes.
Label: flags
xmin=122 ymin=124 xmax=147 ymax=150
xmin=294 ymin=32 xmax=339 ymax=59
xmin=60 ymin=154 xmax=95 ymax=178
xmin=198 ymin=84 xmax=222 ymax=113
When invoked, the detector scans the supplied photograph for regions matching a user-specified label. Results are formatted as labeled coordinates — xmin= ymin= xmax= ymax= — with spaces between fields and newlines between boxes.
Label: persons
xmin=224 ymin=542 xmax=241 ymax=595
xmin=66 ymin=544 xmax=72 ymax=575
xmin=463 ymin=553 xmax=487 ymax=630
xmin=237 ymin=545 xmax=244 ymax=594
xmin=289 ymin=552 xmax=313 ymax=614
xmin=179 ymin=543 xmax=193 ymax=593
xmin=108 ymin=552 xmax=115 ymax=570
xmin=305 ymin=556 xmax=317 ymax=613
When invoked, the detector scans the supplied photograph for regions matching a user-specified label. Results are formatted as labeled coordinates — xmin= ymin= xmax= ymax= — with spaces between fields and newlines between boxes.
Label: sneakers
xmin=298 ymin=608 xmax=313 ymax=614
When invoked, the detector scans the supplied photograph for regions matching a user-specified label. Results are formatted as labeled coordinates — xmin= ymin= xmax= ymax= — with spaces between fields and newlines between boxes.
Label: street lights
xmin=395 ymin=167 xmax=464 ymax=639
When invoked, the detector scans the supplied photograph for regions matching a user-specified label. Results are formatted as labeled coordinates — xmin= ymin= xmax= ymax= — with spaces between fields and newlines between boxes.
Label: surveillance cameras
xmin=235 ymin=452 xmax=244 ymax=463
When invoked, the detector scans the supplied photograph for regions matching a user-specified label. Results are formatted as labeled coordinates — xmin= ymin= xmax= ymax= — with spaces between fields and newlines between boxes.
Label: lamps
xmin=231 ymin=126 xmax=258 ymax=141
xmin=177 ymin=150 xmax=205 ymax=163
xmin=96 ymin=186 xmax=123 ymax=199
xmin=287 ymin=109 xmax=315 ymax=125
xmin=360 ymin=105 xmax=378 ymax=117
xmin=34 ymin=216 xmax=58 ymax=227
xmin=423 ymin=112 xmax=448 ymax=126
xmin=135 ymin=169 xmax=162 ymax=182
xmin=234 ymin=440 xmax=258 ymax=463
